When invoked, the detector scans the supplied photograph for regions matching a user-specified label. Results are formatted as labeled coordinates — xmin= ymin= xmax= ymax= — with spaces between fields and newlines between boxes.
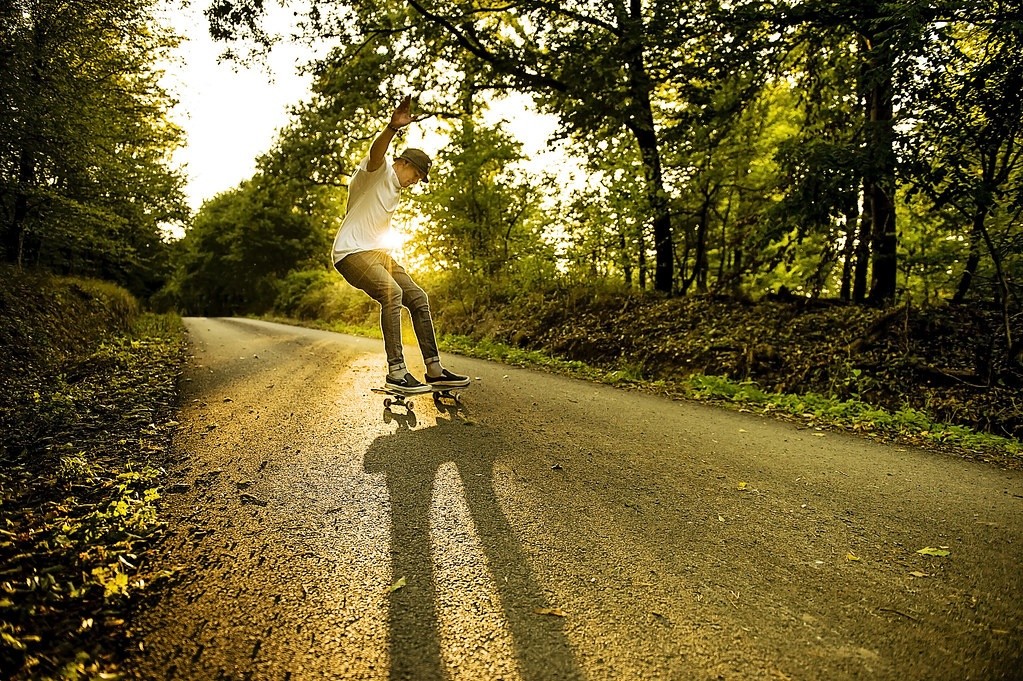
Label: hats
xmin=393 ymin=148 xmax=432 ymax=184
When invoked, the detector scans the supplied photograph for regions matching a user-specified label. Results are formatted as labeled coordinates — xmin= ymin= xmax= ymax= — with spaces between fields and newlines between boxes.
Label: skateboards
xmin=370 ymin=380 xmax=471 ymax=411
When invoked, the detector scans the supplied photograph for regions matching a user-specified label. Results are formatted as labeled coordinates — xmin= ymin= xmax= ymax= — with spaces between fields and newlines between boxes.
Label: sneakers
xmin=385 ymin=372 xmax=432 ymax=393
xmin=424 ymin=369 xmax=470 ymax=386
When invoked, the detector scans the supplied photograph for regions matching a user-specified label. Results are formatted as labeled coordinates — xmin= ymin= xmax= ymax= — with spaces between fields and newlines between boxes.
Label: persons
xmin=331 ymin=93 xmax=471 ymax=394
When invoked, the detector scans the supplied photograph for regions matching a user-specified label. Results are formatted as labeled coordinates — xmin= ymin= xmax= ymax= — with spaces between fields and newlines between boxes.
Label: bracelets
xmin=388 ymin=123 xmax=398 ymax=132
